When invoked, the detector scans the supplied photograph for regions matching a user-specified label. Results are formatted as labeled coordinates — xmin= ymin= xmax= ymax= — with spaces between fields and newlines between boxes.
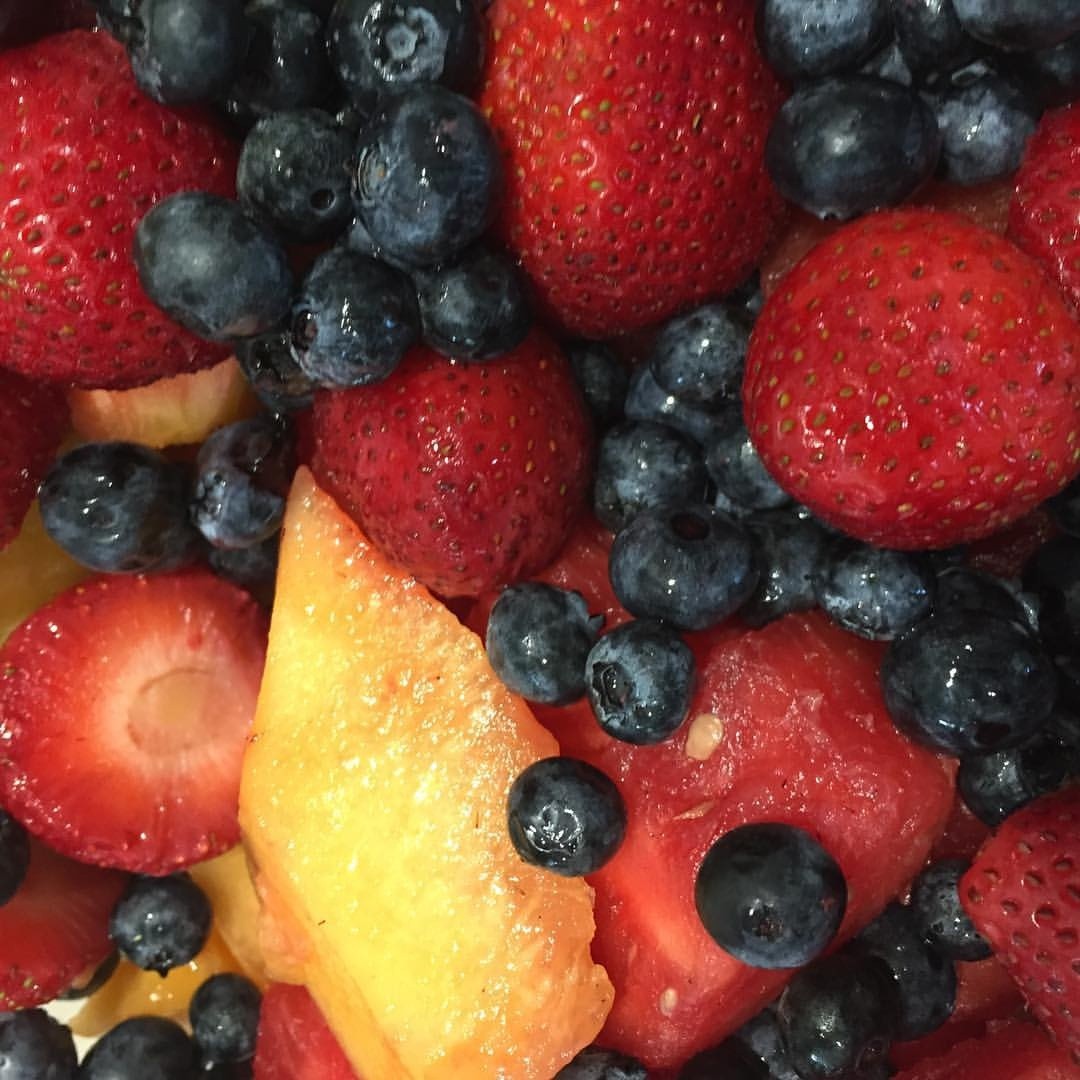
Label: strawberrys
xmin=0 ymin=0 xmax=1080 ymax=1080
